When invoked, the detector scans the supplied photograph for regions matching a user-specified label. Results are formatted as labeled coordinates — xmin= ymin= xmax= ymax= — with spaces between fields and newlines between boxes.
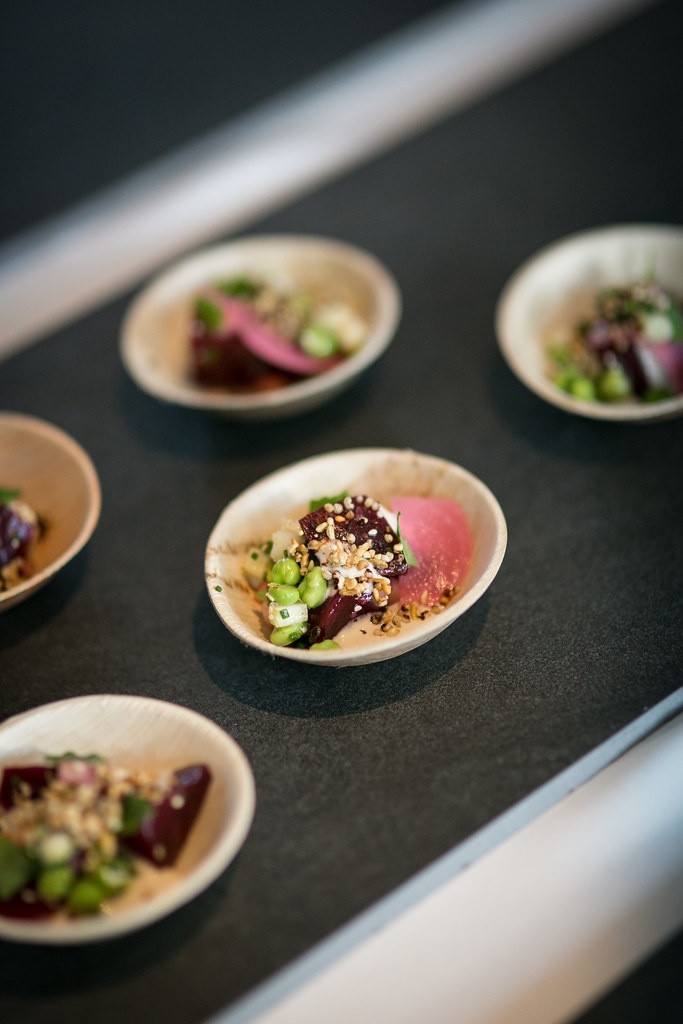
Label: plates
xmin=494 ymin=226 xmax=683 ymax=421
xmin=118 ymin=233 xmax=403 ymax=414
xmin=203 ymin=447 xmax=507 ymax=666
xmin=0 ymin=413 xmax=101 ymax=611
xmin=0 ymin=694 xmax=256 ymax=943
